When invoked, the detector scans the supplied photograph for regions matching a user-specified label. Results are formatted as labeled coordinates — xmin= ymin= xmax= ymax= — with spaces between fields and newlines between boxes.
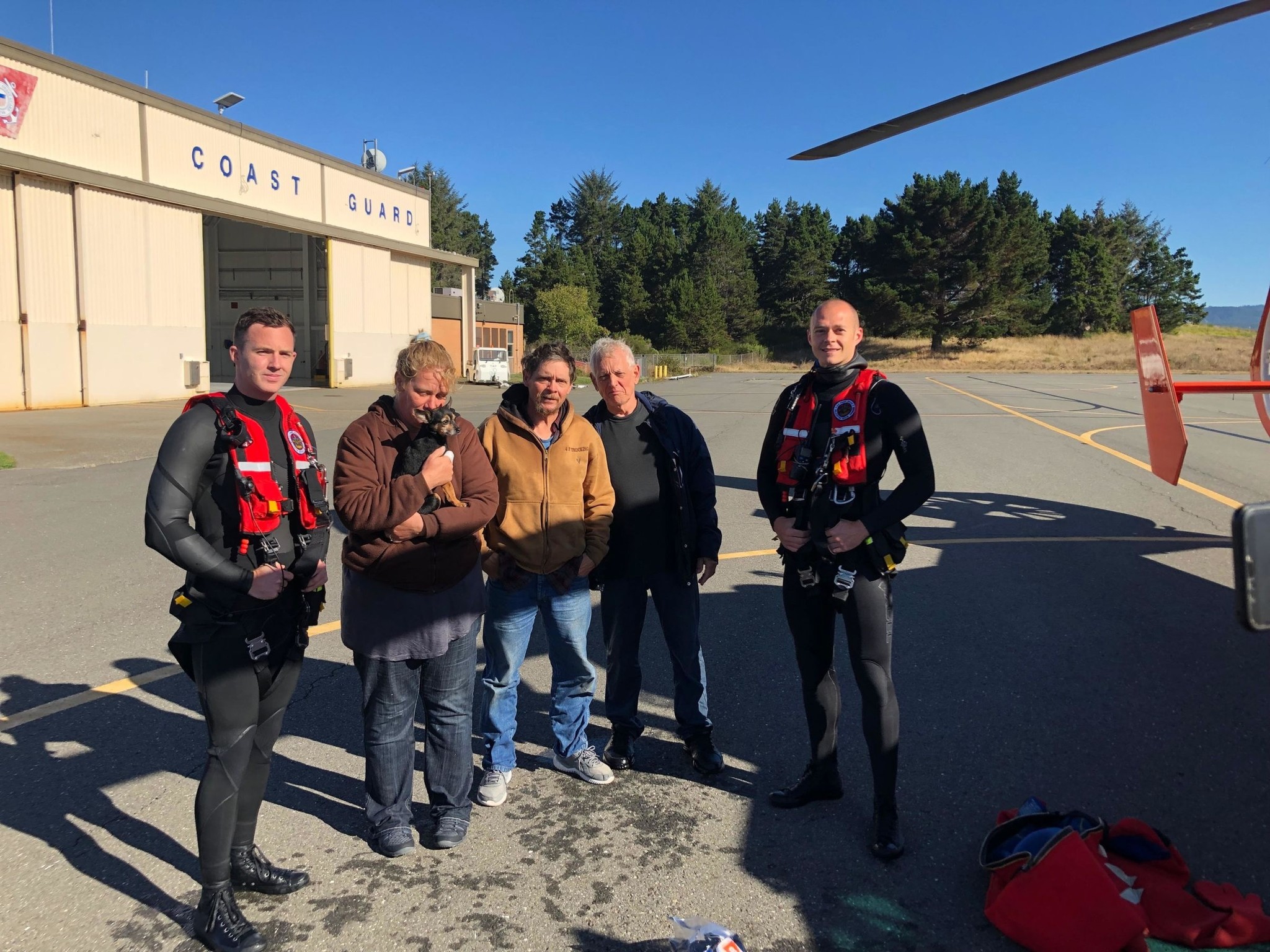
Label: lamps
xmin=212 ymin=92 xmax=244 ymax=109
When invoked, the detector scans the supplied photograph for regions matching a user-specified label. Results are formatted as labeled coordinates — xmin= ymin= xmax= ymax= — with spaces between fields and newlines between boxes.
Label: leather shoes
xmin=683 ymin=737 xmax=724 ymax=775
xmin=192 ymin=883 xmax=265 ymax=952
xmin=769 ymin=764 xmax=844 ymax=810
xmin=871 ymin=807 xmax=905 ymax=861
xmin=232 ymin=843 xmax=309 ymax=893
xmin=602 ymin=731 xmax=634 ymax=770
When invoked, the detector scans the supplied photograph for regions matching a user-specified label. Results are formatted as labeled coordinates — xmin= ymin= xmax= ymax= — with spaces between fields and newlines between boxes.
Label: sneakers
xmin=434 ymin=816 xmax=469 ymax=847
xmin=477 ymin=767 xmax=513 ymax=806
xmin=379 ymin=830 xmax=415 ymax=857
xmin=553 ymin=746 xmax=613 ymax=785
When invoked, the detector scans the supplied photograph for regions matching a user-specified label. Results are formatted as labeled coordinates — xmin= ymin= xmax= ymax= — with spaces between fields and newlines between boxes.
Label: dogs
xmin=390 ymin=397 xmax=468 ymax=515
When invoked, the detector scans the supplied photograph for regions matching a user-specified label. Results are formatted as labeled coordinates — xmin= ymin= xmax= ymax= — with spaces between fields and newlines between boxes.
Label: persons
xmin=144 ymin=306 xmax=334 ymax=952
xmin=580 ymin=338 xmax=726 ymax=776
xmin=332 ymin=329 xmax=499 ymax=857
xmin=757 ymin=299 xmax=936 ymax=859
xmin=473 ymin=343 xmax=614 ymax=807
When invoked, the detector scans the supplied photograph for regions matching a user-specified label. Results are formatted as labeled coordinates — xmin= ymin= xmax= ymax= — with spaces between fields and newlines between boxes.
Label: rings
xmin=389 ymin=534 xmax=393 ymax=541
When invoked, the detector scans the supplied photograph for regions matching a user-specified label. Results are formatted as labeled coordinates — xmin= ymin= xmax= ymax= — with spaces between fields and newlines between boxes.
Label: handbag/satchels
xmin=976 ymin=797 xmax=1270 ymax=952
